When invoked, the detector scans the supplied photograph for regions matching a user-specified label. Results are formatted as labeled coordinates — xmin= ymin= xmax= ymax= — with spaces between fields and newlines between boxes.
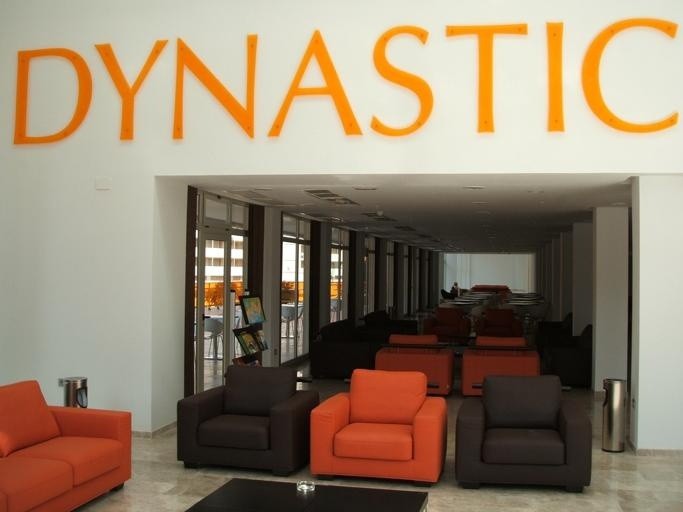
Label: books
xmin=232 ymin=294 xmax=268 ymax=368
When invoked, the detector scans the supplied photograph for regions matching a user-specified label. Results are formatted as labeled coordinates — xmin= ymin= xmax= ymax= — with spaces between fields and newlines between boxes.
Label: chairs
xmin=455 ymin=392 xmax=592 ymax=492
xmin=318 ymin=285 xmax=592 ymax=398
xmin=176 ymin=390 xmax=319 ymax=477
xmin=310 ymin=392 xmax=447 ymax=488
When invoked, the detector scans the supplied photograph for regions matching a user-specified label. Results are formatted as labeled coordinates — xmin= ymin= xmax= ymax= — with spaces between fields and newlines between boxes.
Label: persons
xmin=454 ymin=282 xmax=460 ymax=297
xmin=450 ymin=287 xmax=457 ymax=297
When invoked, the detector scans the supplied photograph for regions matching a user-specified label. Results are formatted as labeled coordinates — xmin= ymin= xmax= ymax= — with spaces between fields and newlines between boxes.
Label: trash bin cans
xmin=63 ymin=377 xmax=88 ymax=408
xmin=601 ymin=378 xmax=626 ymax=452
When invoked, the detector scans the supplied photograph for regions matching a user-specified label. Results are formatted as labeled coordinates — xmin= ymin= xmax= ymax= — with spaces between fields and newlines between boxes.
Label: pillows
xmin=482 ymin=375 xmax=560 ymax=427
xmin=349 ymin=369 xmax=427 ymax=424
xmin=0 ymin=380 xmax=60 ymax=456
xmin=225 ymin=364 xmax=297 ymax=415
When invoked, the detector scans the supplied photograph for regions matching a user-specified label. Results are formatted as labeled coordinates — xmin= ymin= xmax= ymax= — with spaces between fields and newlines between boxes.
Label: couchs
xmin=0 ymin=405 xmax=132 ymax=512
